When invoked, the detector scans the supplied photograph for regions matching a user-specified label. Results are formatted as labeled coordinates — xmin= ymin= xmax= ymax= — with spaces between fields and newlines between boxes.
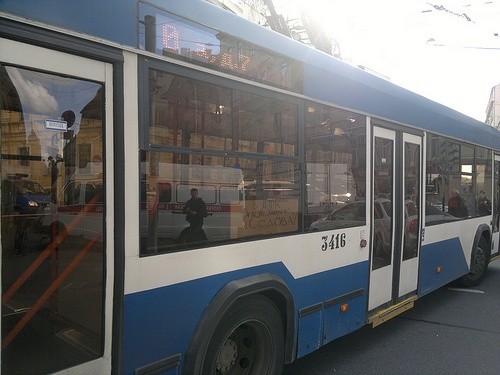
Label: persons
xmin=478 ymin=190 xmax=492 ymax=216
xmin=448 ymin=190 xmax=469 ymax=218
xmin=183 ymin=188 xmax=208 ymax=247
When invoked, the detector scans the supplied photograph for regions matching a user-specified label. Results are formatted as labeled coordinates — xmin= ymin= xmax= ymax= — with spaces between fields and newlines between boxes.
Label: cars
xmin=2 ymin=166 xmax=468 ymax=260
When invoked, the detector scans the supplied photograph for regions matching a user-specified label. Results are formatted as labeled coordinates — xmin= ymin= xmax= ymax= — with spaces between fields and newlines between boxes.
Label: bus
xmin=0 ymin=0 xmax=500 ymax=375
